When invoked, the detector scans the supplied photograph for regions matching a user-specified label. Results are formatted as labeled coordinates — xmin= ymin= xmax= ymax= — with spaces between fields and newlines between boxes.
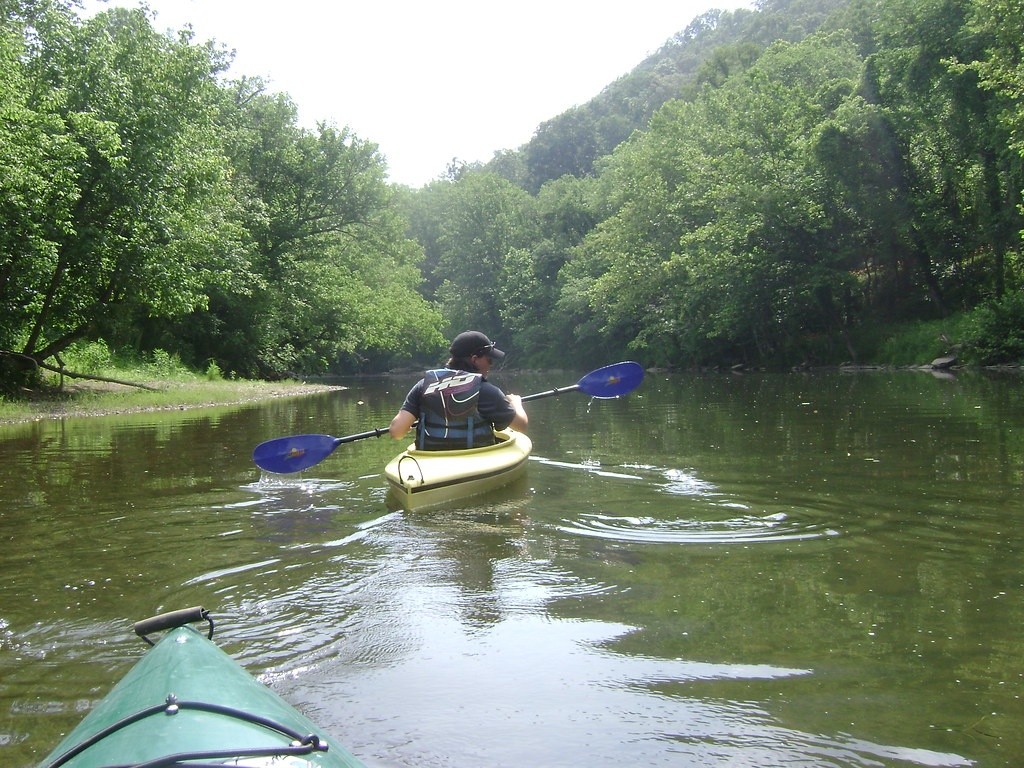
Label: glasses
xmin=470 ymin=341 xmax=496 ymax=357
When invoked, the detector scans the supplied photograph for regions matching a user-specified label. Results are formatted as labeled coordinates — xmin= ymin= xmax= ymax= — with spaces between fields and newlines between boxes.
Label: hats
xmin=449 ymin=330 xmax=505 ymax=360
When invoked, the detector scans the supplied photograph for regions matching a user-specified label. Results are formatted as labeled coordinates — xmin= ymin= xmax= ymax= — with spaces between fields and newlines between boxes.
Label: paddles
xmin=251 ymin=360 xmax=645 ymax=474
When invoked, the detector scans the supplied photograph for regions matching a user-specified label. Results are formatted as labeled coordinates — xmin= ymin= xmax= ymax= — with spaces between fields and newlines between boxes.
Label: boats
xmin=384 ymin=422 xmax=534 ymax=515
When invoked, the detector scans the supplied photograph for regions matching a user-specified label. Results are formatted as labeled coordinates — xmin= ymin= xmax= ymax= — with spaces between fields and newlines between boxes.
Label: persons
xmin=389 ymin=330 xmax=529 ymax=451
